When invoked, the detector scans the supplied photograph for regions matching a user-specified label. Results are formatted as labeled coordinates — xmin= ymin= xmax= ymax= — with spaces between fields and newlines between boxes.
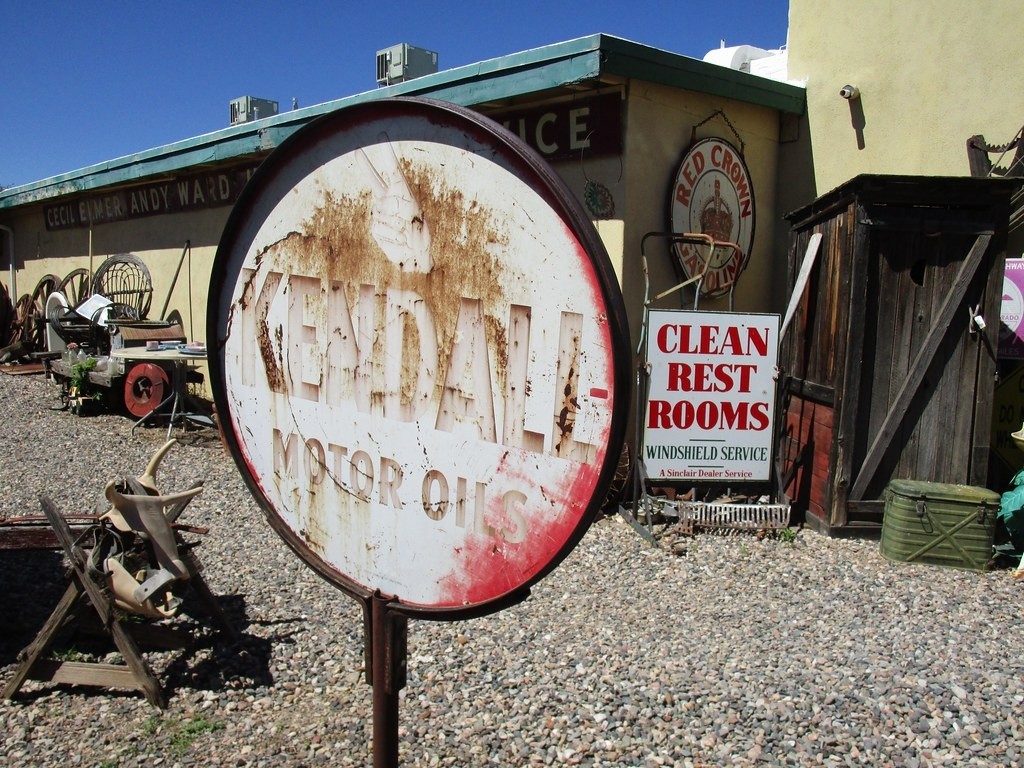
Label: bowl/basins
xmin=161 ymin=341 xmax=181 ymax=346
xmin=185 ymin=347 xmax=206 ymax=352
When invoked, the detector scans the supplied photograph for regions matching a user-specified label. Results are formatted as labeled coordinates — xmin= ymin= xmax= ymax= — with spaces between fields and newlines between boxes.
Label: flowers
xmin=66 ymin=342 xmax=78 ymax=350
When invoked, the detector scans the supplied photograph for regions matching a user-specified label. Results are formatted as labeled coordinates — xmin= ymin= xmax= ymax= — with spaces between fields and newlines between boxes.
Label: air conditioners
xmin=230 ymin=95 xmax=279 ymax=126
xmin=376 ymin=42 xmax=438 ymax=84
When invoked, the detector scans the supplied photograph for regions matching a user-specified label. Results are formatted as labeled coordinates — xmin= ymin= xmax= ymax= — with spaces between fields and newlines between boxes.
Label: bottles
xmin=78 ymin=349 xmax=86 ymax=361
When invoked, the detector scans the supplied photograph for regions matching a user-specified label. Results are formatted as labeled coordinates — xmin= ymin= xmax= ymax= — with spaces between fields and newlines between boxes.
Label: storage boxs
xmin=878 ymin=479 xmax=1001 ymax=574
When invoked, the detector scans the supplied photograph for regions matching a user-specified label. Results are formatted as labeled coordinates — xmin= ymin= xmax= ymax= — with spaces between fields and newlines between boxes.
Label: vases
xmin=69 ymin=349 xmax=77 ymax=365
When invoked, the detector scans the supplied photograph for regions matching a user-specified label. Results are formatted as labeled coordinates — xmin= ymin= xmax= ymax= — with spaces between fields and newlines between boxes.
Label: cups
xmin=147 ymin=342 xmax=158 ymax=349
xmin=192 ymin=341 xmax=204 ymax=346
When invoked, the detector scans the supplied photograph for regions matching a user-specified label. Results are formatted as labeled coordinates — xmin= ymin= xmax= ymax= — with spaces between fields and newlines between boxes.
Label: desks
xmin=111 ymin=344 xmax=207 ymax=443
